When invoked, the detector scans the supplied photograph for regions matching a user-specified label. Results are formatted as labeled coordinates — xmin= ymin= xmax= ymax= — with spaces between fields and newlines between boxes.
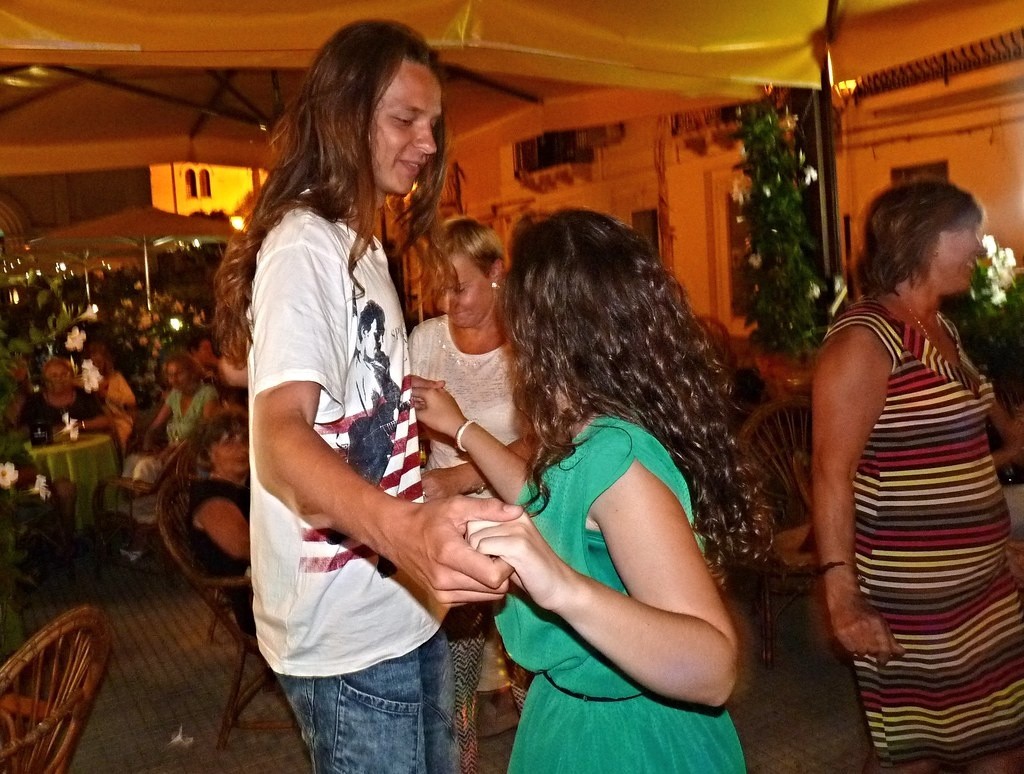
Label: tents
xmin=0 ymin=0 xmax=1024 ymax=318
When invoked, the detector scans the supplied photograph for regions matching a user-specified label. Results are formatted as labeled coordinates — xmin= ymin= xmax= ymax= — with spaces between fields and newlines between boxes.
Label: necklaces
xmin=907 ymin=306 xmax=932 ymax=342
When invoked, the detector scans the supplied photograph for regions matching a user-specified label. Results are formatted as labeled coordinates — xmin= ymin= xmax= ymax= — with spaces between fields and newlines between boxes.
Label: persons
xmin=812 ymin=180 xmax=1024 ymax=774
xmin=0 ymin=313 xmax=254 ymax=634
xmin=413 ymin=208 xmax=774 ymax=774
xmin=408 ymin=216 xmax=541 ymax=774
xmin=215 ymin=18 xmax=518 ymax=774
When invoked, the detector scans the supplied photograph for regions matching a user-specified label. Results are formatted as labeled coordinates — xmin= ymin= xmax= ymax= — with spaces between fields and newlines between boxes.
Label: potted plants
xmin=732 ymin=94 xmax=839 ymax=404
xmin=934 ymin=234 xmax=1024 ymax=490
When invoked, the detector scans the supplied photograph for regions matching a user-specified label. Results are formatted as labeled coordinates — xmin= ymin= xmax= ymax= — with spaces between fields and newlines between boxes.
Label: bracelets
xmin=477 ymin=482 xmax=488 ymax=494
xmin=822 ymin=561 xmax=854 ymax=574
xmin=456 ymin=419 xmax=475 ymax=452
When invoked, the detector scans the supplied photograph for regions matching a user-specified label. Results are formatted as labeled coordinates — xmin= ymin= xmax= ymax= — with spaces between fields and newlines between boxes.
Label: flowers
xmin=108 ymin=282 xmax=206 ymax=395
xmin=1 ymin=270 xmax=103 ymax=436
xmin=50 ymin=414 xmax=80 ymax=443
xmin=1 ymin=442 xmax=52 ymax=659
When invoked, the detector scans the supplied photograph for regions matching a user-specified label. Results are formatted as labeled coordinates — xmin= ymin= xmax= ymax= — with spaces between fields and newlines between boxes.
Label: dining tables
xmin=24 ymin=430 xmax=123 ymax=551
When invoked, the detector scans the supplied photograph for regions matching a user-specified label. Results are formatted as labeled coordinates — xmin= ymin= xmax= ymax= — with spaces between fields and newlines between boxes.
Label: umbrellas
xmin=27 ymin=199 xmax=239 ymax=310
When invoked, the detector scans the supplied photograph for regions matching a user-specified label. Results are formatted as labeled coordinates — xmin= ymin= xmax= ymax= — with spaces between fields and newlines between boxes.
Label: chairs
xmin=738 ymin=395 xmax=819 ymax=670
xmin=157 ymin=469 xmax=306 ymax=753
xmin=93 ymin=438 xmax=194 ymax=560
xmin=1 ymin=601 xmax=110 ymax=773
